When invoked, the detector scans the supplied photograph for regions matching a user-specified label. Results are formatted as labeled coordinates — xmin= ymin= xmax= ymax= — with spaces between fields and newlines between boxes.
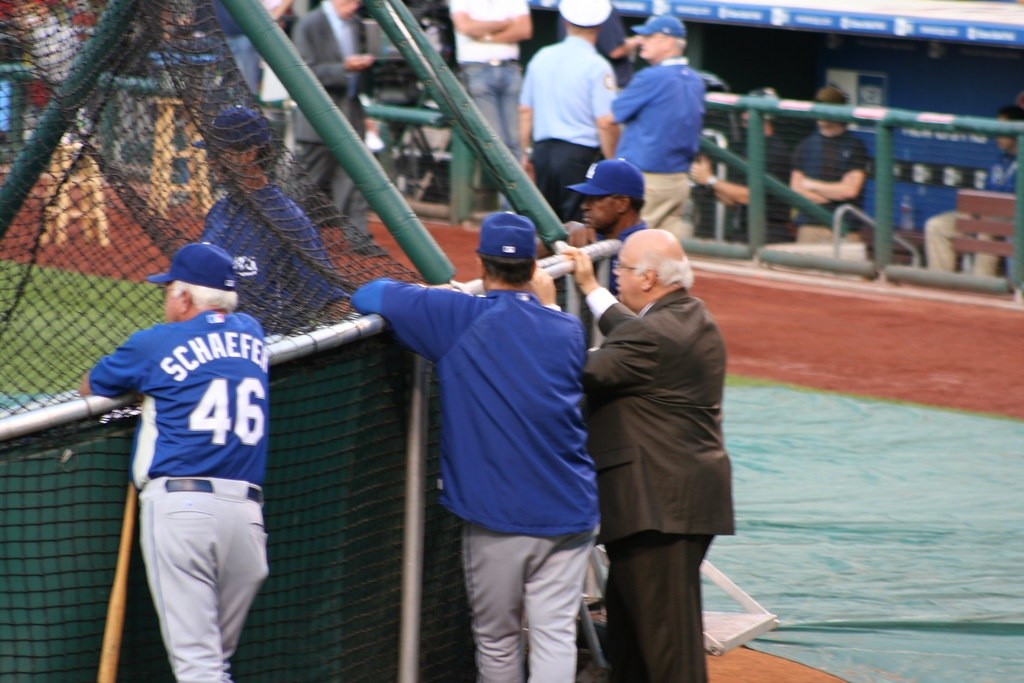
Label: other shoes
xmin=352 ymin=240 xmax=390 ymax=257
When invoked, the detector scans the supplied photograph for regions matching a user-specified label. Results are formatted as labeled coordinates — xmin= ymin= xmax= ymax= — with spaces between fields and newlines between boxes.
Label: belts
xmin=167 ymin=480 xmax=260 ymax=503
xmin=470 ymin=59 xmax=519 ymax=69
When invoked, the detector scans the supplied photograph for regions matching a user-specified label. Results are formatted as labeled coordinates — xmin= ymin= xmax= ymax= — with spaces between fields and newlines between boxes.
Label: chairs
xmin=392 ymin=97 xmax=452 ymax=202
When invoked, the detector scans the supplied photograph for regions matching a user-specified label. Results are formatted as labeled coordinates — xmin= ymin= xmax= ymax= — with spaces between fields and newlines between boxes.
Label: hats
xmin=478 ymin=211 xmax=538 ymax=258
xmin=558 ymin=0 xmax=613 ymax=27
xmin=631 ymin=15 xmax=686 ymax=38
xmin=191 ymin=104 xmax=271 ymax=149
xmin=565 ymin=156 xmax=645 ymax=200
xmin=146 ymin=242 xmax=237 ymax=292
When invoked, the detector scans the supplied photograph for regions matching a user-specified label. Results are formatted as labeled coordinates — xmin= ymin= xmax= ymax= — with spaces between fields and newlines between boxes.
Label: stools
xmin=147 ymin=96 xmax=215 ymax=218
xmin=38 ymin=141 xmax=112 ymax=248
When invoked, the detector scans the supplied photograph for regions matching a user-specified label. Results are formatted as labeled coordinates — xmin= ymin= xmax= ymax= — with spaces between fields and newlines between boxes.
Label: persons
xmin=527 ymin=229 xmax=737 ymax=683
xmin=448 ymin=0 xmax=532 ymax=211
xmin=606 ymin=15 xmax=705 ymax=242
xmin=556 ymin=0 xmax=645 ymax=89
xmin=565 ymin=157 xmax=650 ymax=611
xmin=349 ymin=211 xmax=600 ymax=683
xmin=79 ymin=242 xmax=270 ymax=683
xmin=191 ymin=105 xmax=355 ymax=342
xmin=518 ymin=0 xmax=621 ymax=224
xmin=790 ymin=85 xmax=870 ymax=243
xmin=167 ymin=0 xmax=386 ymax=257
xmin=689 ymin=88 xmax=792 ymax=245
xmin=924 ymin=104 xmax=1024 ymax=279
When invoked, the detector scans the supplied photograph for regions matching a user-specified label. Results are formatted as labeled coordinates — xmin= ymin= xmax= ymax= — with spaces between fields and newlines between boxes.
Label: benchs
xmin=950 ymin=188 xmax=1018 ymax=259
xmin=857 ymin=223 xmax=924 ymax=265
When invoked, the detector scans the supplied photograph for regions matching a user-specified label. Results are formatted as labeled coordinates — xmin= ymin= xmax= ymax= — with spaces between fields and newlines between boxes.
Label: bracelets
xmin=484 ymin=32 xmax=494 ymax=43
xmin=707 ymin=177 xmax=719 ymax=187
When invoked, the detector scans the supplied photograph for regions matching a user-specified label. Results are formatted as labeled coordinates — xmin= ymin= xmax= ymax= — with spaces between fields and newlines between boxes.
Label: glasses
xmin=161 ymin=286 xmax=183 ymax=298
xmin=613 ymin=260 xmax=659 ymax=277
